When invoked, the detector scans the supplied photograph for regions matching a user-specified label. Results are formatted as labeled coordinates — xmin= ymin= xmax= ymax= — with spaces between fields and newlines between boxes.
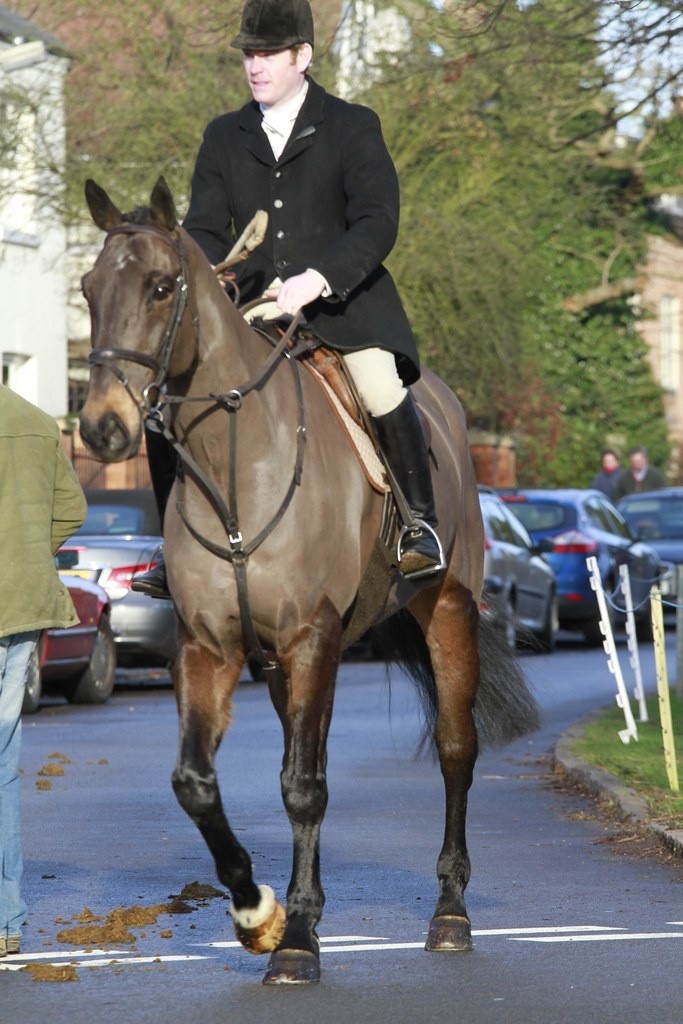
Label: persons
xmin=0 ymin=380 xmax=88 ymax=960
xmin=180 ymin=0 xmax=440 ymax=576
xmin=589 ymin=446 xmax=666 ymax=502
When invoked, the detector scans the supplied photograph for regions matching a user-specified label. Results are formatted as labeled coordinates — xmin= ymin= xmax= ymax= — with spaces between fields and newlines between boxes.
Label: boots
xmin=371 ymin=390 xmax=440 ymax=581
xmin=131 ymin=402 xmax=172 ymax=598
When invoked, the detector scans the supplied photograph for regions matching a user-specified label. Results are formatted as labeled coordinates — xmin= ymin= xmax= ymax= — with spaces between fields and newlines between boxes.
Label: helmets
xmin=230 ymin=0 xmax=314 ymax=51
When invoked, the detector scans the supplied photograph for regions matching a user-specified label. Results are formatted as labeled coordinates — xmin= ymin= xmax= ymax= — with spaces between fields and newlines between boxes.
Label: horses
xmin=80 ymin=174 xmax=542 ymax=985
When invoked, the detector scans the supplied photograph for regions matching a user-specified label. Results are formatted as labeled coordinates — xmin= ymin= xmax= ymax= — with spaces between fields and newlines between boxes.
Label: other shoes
xmin=0 ymin=932 xmax=23 ymax=957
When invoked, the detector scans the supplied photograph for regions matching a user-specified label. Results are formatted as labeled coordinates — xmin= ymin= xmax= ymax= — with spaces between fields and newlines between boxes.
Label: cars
xmin=21 ymin=499 xmax=267 ymax=717
xmin=612 ymin=486 xmax=683 ymax=630
xmin=497 ymin=486 xmax=665 ymax=648
xmin=474 ymin=482 xmax=560 ymax=656
